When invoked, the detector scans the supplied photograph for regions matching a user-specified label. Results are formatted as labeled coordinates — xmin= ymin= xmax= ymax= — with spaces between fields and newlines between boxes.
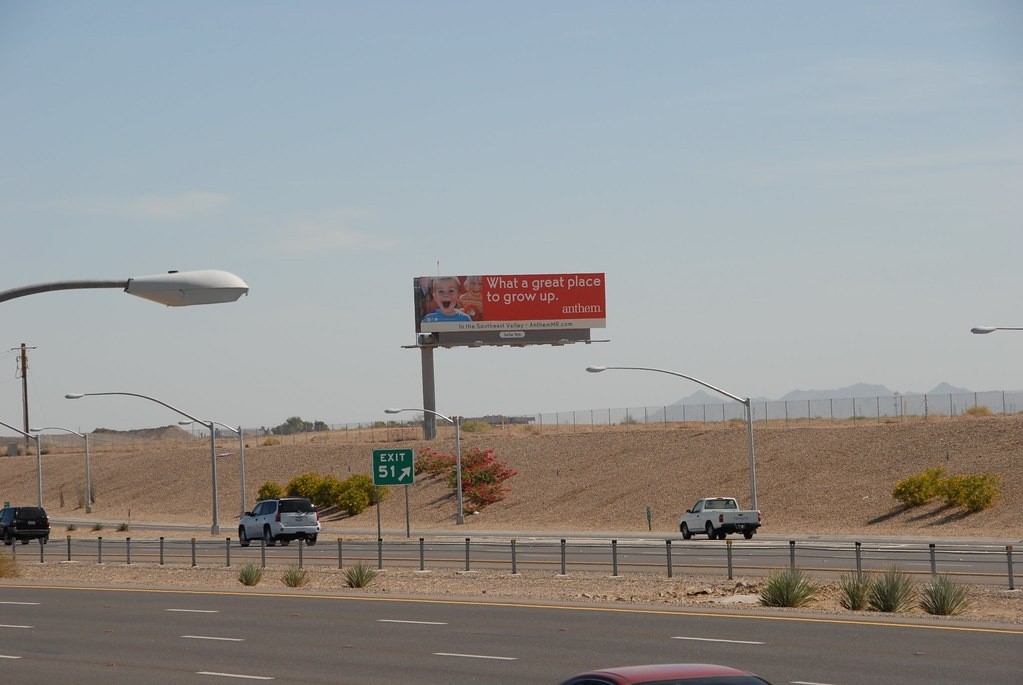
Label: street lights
xmin=64 ymin=393 xmax=221 ymax=536
xmin=383 ymin=408 xmax=464 ymax=526
xmin=29 ymin=427 xmax=92 ymax=515
xmin=587 ymin=365 xmax=758 ymax=510
xmin=177 ymin=419 xmax=245 ymax=514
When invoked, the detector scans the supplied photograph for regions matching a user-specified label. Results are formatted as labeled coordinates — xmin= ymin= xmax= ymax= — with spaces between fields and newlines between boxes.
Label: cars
xmin=557 ymin=664 xmax=771 ymax=685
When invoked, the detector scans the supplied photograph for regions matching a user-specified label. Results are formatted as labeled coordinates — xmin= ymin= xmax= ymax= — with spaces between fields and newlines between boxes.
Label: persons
xmin=422 ymin=277 xmax=482 ymax=321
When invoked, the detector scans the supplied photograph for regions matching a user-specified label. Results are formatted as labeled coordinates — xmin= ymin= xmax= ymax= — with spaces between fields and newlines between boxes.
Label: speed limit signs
xmin=372 ymin=448 xmax=416 ymax=487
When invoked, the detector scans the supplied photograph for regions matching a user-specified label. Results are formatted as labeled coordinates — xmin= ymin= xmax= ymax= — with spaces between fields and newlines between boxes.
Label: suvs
xmin=238 ymin=497 xmax=321 ymax=547
xmin=0 ymin=506 xmax=51 ymax=546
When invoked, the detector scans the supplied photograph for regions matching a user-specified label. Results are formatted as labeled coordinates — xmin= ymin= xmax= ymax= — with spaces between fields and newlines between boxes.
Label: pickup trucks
xmin=681 ymin=500 xmax=763 ymax=541
